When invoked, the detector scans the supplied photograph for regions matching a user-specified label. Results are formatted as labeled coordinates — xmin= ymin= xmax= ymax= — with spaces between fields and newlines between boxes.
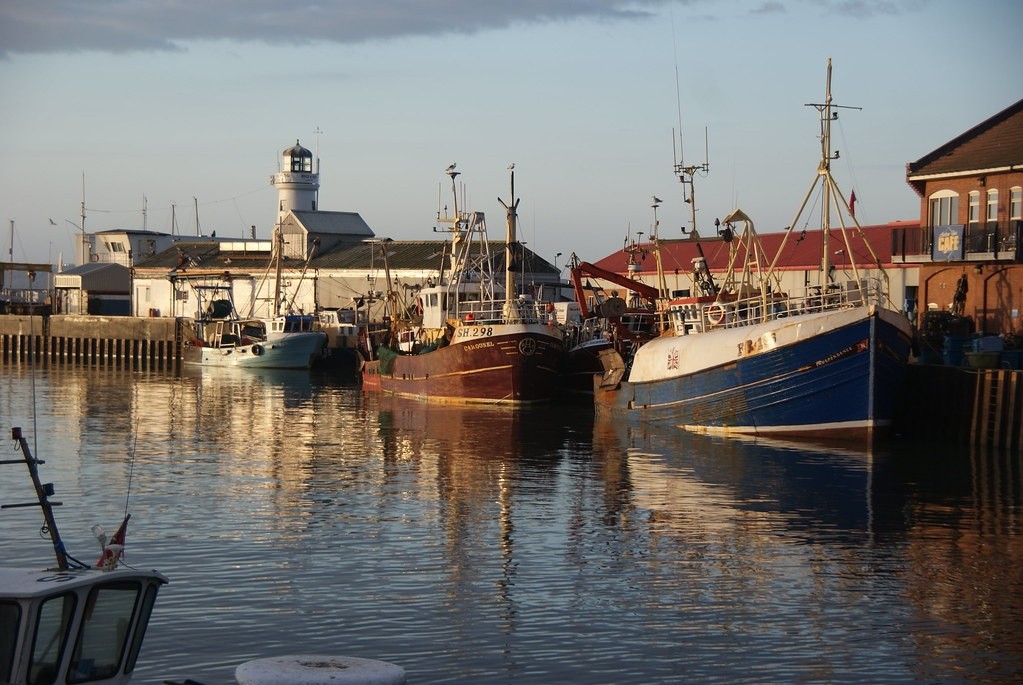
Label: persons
xmin=548 ymin=302 xmax=553 ymax=313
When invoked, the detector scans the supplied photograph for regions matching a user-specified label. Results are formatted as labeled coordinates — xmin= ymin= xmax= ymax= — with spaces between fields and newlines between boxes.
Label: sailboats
xmin=1 ymin=219 xmax=51 ymax=316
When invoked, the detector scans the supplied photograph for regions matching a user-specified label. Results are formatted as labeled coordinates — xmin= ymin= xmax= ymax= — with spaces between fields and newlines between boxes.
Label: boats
xmin=360 ymin=161 xmax=566 ymax=406
xmin=567 ymin=49 xmax=917 ymax=447
xmin=181 ymin=213 xmax=389 ymax=371
xmin=0 ymin=299 xmax=209 ymax=685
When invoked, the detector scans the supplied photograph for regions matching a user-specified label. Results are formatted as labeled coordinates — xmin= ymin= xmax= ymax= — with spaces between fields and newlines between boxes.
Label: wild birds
xmin=445 ymin=162 xmax=456 ymax=171
xmin=507 ymin=163 xmax=515 ymax=169
xmin=651 ymin=195 xmax=663 ymax=203
xmin=49 ymin=218 xmax=57 ymax=225
xmin=211 ymin=230 xmax=215 ymax=241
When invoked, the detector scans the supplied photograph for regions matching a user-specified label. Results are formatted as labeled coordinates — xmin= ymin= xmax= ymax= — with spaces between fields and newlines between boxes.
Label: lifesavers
xmin=707 ymin=301 xmax=725 ymax=324
xmin=251 ymin=345 xmax=260 ymax=356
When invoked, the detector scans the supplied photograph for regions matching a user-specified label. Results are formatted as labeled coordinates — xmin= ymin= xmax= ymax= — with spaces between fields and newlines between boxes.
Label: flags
xmin=849 ymin=189 xmax=857 ymax=216
